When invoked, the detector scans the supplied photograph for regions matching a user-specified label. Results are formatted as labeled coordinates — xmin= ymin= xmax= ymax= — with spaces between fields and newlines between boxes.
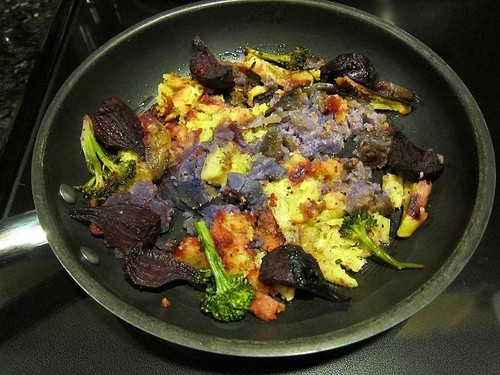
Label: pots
xmin=0 ymin=0 xmax=496 ymax=359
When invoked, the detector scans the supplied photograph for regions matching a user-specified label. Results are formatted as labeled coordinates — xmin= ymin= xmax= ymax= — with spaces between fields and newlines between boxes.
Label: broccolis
xmin=72 ymin=113 xmax=136 ymax=200
xmin=338 ymin=210 xmax=426 ymax=268
xmin=195 ymin=220 xmax=255 ymax=323
xmin=244 ymin=46 xmax=312 ymax=69
